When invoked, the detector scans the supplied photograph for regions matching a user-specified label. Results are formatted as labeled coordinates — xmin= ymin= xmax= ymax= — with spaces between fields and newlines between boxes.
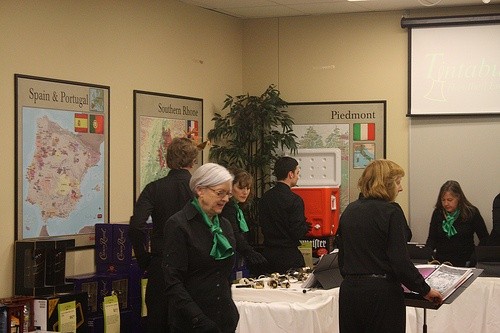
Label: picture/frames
xmin=133 ymin=89 xmax=204 ymax=215
xmin=261 ymin=100 xmax=387 ymax=218
xmin=14 ymin=73 xmax=110 ymax=252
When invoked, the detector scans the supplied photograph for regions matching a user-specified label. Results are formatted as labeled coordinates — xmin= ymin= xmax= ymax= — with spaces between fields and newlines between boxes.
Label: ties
xmin=442 ymin=210 xmax=457 ymax=237
xmin=231 ymin=200 xmax=250 ymax=234
xmin=192 ymin=197 xmax=235 ymax=260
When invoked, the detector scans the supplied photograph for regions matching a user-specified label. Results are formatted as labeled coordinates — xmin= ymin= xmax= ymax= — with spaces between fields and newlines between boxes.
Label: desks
xmin=233 ymin=273 xmax=500 ymax=333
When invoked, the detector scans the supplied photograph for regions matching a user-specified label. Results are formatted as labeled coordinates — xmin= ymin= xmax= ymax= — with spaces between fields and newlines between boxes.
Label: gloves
xmin=246 ymin=250 xmax=266 ymax=264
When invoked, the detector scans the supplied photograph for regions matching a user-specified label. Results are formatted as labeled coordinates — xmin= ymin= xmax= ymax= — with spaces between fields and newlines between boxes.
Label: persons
xmin=132 ymin=137 xmax=199 ymax=333
xmin=215 ymin=167 xmax=265 ymax=281
xmin=336 ymin=159 xmax=441 ymax=333
xmin=425 ymin=180 xmax=489 ymax=268
xmin=257 ymin=157 xmax=315 ymax=275
xmin=162 ymin=162 xmax=240 ymax=333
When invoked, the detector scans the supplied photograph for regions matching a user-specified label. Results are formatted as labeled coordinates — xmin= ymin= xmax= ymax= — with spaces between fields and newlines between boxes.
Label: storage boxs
xmin=304 ymin=236 xmax=337 ymax=259
xmin=0 ymin=222 xmax=152 ymax=333
xmin=285 ymin=147 xmax=341 ymax=237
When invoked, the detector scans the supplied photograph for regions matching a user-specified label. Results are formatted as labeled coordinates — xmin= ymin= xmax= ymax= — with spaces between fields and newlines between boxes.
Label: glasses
xmin=208 ymin=186 xmax=233 ymax=199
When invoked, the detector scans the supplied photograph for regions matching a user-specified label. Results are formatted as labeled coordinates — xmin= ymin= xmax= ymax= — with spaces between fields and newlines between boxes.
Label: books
xmin=399 ymin=263 xmax=473 ymax=301
